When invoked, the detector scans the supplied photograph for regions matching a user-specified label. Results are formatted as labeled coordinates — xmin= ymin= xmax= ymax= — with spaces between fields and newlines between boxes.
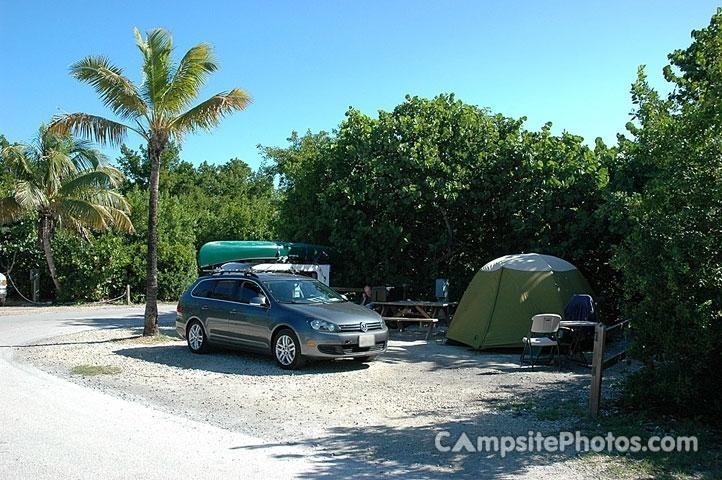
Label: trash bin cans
xmin=17 ymin=269 xmax=40 ymax=304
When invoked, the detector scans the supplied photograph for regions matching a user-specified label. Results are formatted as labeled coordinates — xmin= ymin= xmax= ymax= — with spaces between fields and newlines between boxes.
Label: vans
xmin=199 ymin=239 xmax=344 ymax=295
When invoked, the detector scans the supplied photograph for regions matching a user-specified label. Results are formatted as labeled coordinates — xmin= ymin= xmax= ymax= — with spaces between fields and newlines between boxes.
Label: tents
xmin=445 ymin=252 xmax=596 ymax=354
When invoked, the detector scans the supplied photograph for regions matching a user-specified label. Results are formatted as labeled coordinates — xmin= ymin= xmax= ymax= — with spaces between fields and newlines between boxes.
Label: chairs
xmin=371 ymin=286 xmax=386 ymax=317
xmin=520 ymin=293 xmax=598 ymax=371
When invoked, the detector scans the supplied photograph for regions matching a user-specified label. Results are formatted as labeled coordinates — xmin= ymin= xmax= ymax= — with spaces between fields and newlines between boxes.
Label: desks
xmin=371 ymin=301 xmax=458 ymax=340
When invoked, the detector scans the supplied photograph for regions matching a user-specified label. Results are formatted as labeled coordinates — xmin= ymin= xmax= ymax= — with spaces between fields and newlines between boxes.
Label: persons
xmin=435 ymin=271 xmax=452 ymax=321
xmin=360 ymin=286 xmax=371 ymax=306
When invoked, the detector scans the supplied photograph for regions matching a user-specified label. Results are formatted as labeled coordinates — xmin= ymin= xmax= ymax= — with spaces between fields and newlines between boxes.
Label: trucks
xmin=252 ymin=263 xmax=395 ymax=304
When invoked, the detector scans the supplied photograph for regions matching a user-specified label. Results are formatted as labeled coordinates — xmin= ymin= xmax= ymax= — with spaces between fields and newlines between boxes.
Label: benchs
xmin=383 ymin=317 xmax=439 ymax=340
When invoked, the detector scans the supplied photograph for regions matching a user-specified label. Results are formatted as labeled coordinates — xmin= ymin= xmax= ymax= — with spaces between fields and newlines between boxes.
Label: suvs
xmin=176 ymin=271 xmax=389 ymax=369
